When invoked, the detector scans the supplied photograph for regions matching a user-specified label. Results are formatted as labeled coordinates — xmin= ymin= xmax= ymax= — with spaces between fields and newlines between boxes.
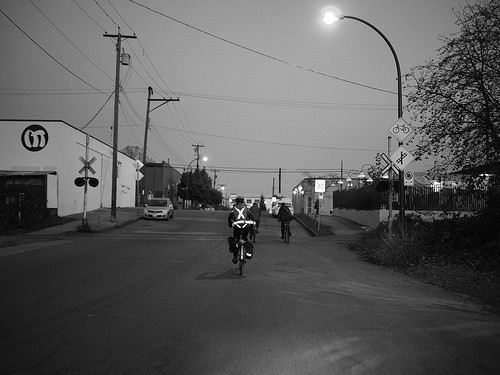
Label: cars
xmin=272 ymin=202 xmax=294 ymax=219
xmin=143 ymin=198 xmax=174 ymax=221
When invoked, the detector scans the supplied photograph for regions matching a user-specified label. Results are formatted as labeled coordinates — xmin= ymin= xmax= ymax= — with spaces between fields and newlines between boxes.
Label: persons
xmin=249 ymin=203 xmax=261 ymax=239
xmin=278 ymin=203 xmax=291 ymax=240
xmin=228 ymin=196 xmax=254 ymax=264
xmin=315 ymin=199 xmax=319 ymax=214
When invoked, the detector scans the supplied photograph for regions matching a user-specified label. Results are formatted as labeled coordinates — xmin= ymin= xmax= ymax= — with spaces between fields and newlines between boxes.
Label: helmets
xmin=236 ymin=196 xmax=244 ymax=200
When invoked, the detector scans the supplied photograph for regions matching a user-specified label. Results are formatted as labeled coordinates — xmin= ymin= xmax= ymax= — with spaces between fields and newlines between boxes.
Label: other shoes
xmin=232 ymin=258 xmax=237 ymax=264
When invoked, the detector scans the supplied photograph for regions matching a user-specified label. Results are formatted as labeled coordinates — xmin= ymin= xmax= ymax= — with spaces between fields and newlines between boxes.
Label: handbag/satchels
xmin=244 ymin=241 xmax=254 ymax=259
xmin=227 ymin=236 xmax=239 ymax=252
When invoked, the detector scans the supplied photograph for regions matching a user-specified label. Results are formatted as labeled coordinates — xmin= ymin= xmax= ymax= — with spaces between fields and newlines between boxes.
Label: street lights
xmin=318 ymin=2 xmax=404 ymax=227
xmin=186 ymin=157 xmax=208 ymax=209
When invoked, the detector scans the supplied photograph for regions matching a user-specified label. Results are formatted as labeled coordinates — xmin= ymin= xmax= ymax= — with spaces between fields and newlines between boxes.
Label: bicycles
xmin=283 ymin=221 xmax=289 ymax=243
xmin=234 ymin=230 xmax=247 ymax=275
xmin=252 ymin=222 xmax=256 ymax=243
